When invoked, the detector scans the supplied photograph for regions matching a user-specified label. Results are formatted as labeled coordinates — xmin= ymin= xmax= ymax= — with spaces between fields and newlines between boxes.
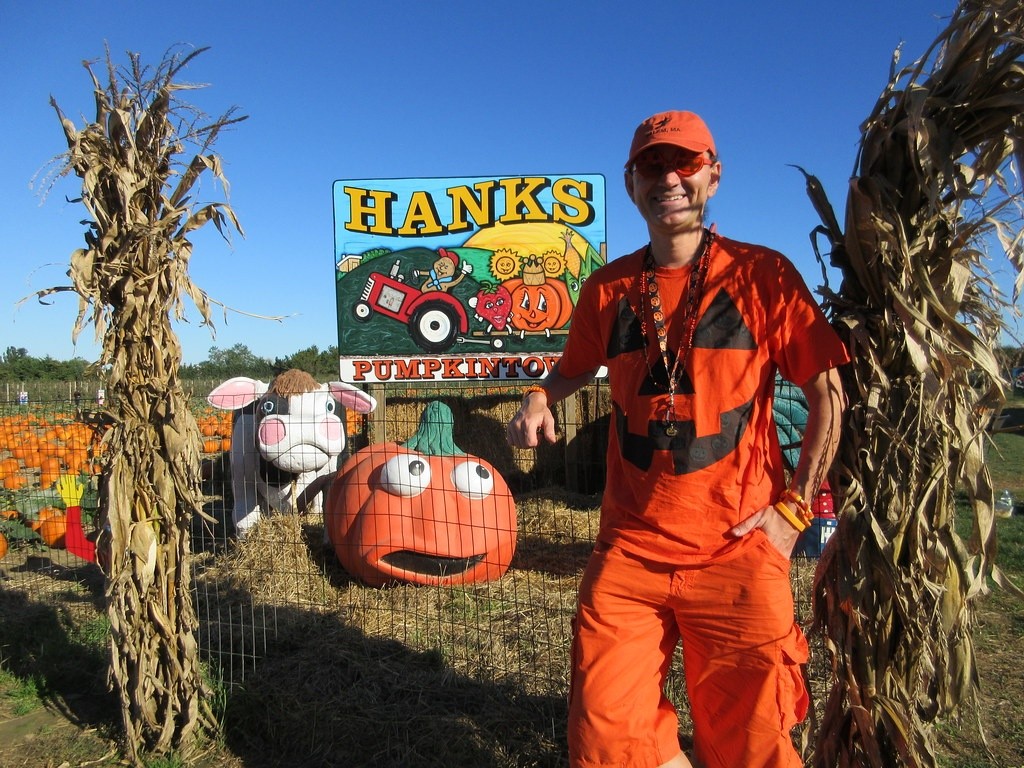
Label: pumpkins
xmin=1 ymin=408 xmax=360 ymax=558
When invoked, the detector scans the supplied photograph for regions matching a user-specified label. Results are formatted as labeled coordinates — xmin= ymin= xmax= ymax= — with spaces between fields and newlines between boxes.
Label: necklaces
xmin=638 ymin=229 xmax=714 ymax=438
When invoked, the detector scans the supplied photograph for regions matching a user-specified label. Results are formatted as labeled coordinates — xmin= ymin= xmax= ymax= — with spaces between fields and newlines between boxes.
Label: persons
xmin=506 ymin=108 xmax=851 ymax=768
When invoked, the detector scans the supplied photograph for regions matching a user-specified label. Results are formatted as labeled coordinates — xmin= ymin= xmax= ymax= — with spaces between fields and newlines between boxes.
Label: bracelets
xmin=523 ymin=382 xmax=556 ymax=409
xmin=774 ymin=489 xmax=815 ymax=532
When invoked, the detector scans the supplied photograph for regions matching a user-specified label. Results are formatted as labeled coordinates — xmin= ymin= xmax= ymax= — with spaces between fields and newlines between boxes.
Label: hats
xmin=624 ymin=110 xmax=717 ymax=170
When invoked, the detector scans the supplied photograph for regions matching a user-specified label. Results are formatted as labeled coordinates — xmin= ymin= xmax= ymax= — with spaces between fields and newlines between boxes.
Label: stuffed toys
xmin=205 ymin=367 xmax=377 ymax=532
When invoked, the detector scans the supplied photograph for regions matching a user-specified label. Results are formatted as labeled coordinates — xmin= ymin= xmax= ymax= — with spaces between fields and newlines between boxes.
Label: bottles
xmin=1000 ymin=489 xmax=1012 ymax=505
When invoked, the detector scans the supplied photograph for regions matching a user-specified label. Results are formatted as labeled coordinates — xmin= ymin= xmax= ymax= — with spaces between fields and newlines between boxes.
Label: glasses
xmin=631 ymin=153 xmax=714 ymax=176
